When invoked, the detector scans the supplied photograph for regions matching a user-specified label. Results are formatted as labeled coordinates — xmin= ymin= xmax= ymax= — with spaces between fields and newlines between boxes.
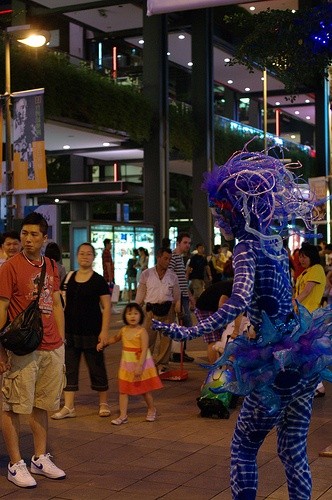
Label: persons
xmin=125 ymin=248 xmax=149 ymax=304
xmin=100 ymin=239 xmax=120 ymax=315
xmin=13 ymin=97 xmax=38 ymax=180
xmin=152 ymin=136 xmax=332 ymax=500
xmin=97 ymin=303 xmax=163 ymax=425
xmin=0 ymin=213 xmax=65 ymax=488
xmin=304 ymin=141 xmax=316 ymax=158
xmin=45 ymin=242 xmax=66 ymax=284
xmin=50 ymin=243 xmax=113 ymax=419
xmin=0 ymin=230 xmax=24 ymax=267
xmin=136 ymin=232 xmax=247 ymax=380
xmin=282 ymin=238 xmax=332 ymax=398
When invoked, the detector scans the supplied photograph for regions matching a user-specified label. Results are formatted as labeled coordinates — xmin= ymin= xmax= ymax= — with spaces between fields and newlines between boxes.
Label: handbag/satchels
xmin=108 ymin=281 xmax=121 ymax=303
xmin=0 ymin=299 xmax=43 ymax=356
xmin=145 ymin=300 xmax=172 ymax=316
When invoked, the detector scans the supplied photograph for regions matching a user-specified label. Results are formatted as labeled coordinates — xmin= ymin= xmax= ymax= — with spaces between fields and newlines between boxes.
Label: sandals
xmin=97 ymin=402 xmax=111 ymax=418
xmin=49 ymin=405 xmax=76 ymax=420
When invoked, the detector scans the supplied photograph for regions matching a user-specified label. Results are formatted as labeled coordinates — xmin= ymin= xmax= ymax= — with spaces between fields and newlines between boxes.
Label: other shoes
xmin=110 ymin=416 xmax=129 ymax=424
xmin=173 ymin=352 xmax=195 ymax=361
xmin=145 ymin=412 xmax=156 ymax=422
xmin=318 ymin=448 xmax=332 ymax=457
xmin=313 ymin=389 xmax=326 ymax=398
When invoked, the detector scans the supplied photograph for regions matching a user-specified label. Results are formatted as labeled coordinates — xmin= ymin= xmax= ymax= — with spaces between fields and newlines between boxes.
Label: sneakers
xmin=30 ymin=452 xmax=67 ymax=480
xmin=8 ymin=459 xmax=38 ymax=489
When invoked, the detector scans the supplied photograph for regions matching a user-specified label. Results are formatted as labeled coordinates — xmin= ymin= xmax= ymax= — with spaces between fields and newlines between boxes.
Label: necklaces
xmin=23 ymin=250 xmax=44 ymax=267
